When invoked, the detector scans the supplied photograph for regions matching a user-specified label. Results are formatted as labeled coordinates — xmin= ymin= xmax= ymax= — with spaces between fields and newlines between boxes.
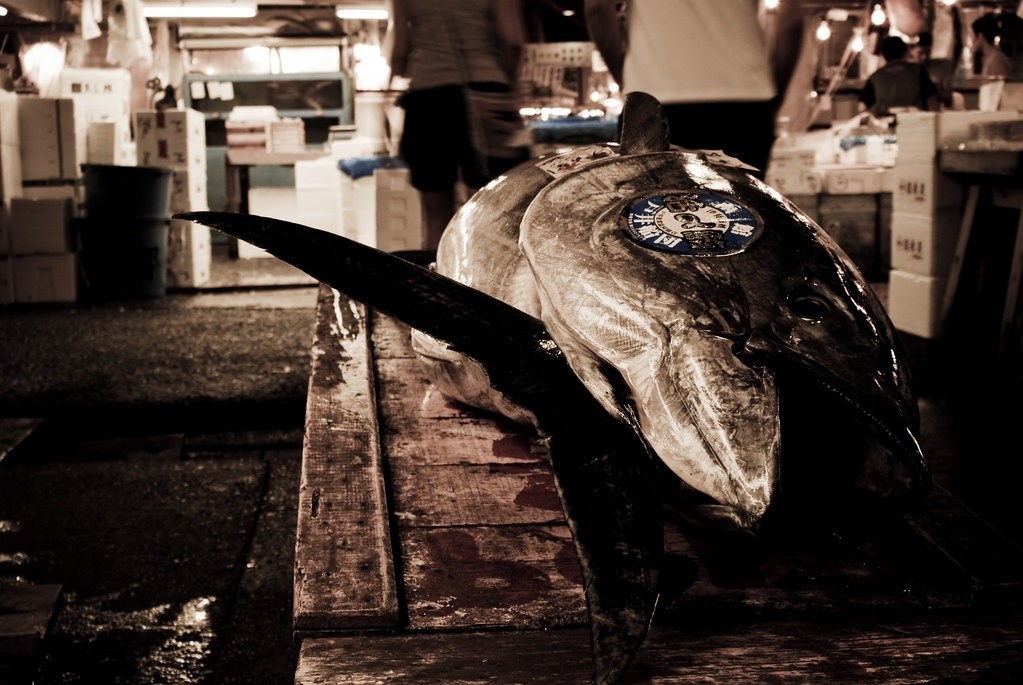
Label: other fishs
xmin=410 ymin=90 xmax=929 ymax=537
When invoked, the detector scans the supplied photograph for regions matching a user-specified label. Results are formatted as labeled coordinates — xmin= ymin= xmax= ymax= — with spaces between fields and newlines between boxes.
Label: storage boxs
xmin=0 ymin=0 xmax=624 ymax=307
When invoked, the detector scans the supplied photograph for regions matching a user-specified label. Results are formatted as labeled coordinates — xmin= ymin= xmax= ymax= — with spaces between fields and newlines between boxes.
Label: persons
xmin=971 ymin=15 xmax=1013 ymax=77
xmin=582 ymin=1 xmax=805 ymax=184
xmin=857 ymin=35 xmax=935 ymax=118
xmin=910 ymin=31 xmax=934 ymax=64
xmin=384 ymin=1 xmax=527 ymax=249
